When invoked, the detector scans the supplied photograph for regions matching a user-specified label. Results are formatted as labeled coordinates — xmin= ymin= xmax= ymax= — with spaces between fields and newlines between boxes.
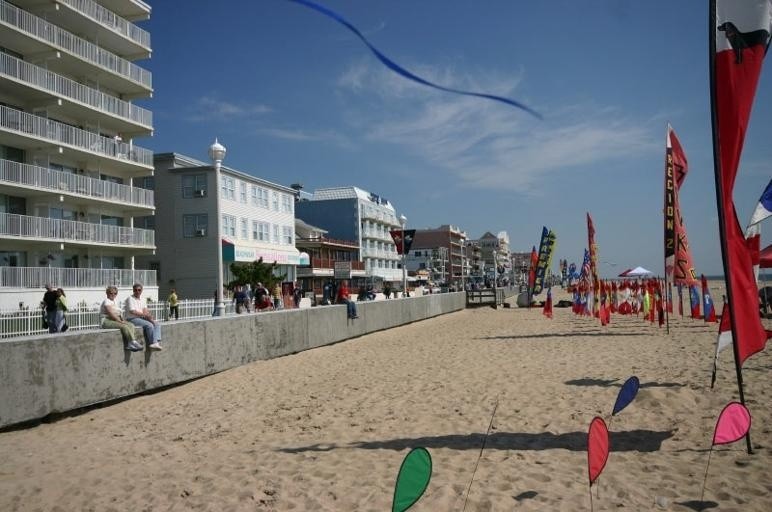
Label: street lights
xmin=492 ymin=249 xmax=497 ymax=289
xmin=209 ymin=136 xmax=228 ymax=316
xmin=399 ymin=213 xmax=409 ymax=298
xmin=458 ymin=238 xmax=465 ymax=291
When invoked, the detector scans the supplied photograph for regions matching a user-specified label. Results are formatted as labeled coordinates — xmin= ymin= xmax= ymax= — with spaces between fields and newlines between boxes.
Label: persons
xmin=385 ymin=284 xmax=391 ymax=299
xmin=167 ymin=288 xmax=179 ymax=319
xmin=211 ymin=279 xmax=375 ymax=319
xmin=98 ymin=284 xmax=163 ymax=352
xmin=41 ymin=283 xmax=68 ymax=333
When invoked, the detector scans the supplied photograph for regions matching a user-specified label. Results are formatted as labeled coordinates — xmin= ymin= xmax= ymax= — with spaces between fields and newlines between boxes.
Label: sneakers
xmin=125 ymin=340 xmax=143 ymax=352
xmin=348 ymin=315 xmax=358 ymax=319
xmin=149 ymin=343 xmax=162 ymax=350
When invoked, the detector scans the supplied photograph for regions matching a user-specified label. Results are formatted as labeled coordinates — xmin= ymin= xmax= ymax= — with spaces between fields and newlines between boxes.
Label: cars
xmin=420 ymin=284 xmax=441 ymax=295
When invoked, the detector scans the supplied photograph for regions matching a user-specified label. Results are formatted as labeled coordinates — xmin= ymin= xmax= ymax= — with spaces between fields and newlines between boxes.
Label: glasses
xmin=134 ymin=289 xmax=141 ymax=291
xmin=110 ymin=293 xmax=117 ymax=294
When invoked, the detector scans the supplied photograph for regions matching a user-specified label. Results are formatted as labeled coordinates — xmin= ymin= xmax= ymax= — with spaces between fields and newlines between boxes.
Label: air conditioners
xmin=196 ymin=229 xmax=204 ymax=236
xmin=194 ymin=190 xmax=205 ymax=197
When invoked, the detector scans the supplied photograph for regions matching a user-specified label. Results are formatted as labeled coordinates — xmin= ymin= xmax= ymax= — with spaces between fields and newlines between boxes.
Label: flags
xmin=543 ymin=272 xmax=732 ymax=348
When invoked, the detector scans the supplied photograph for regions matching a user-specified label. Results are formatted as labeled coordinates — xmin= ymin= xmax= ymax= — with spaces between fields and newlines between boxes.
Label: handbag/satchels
xmin=42 ymin=321 xmax=48 ymax=329
xmin=61 ymin=324 xmax=68 ymax=332
